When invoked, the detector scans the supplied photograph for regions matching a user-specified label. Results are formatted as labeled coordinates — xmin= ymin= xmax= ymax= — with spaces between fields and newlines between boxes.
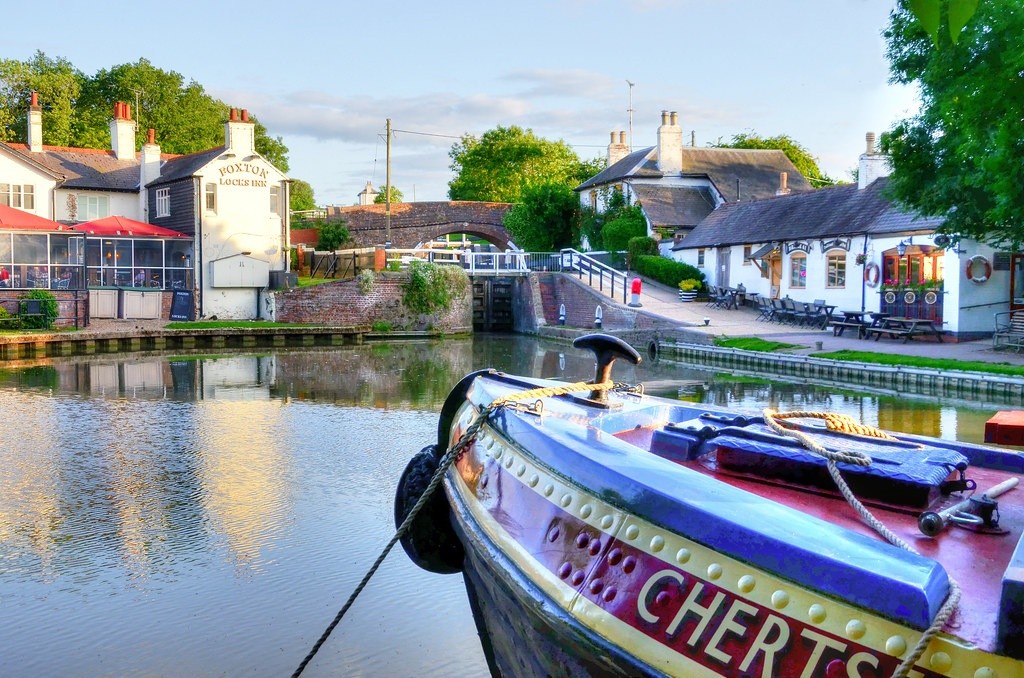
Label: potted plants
xmin=678 ymin=279 xmax=701 ymax=301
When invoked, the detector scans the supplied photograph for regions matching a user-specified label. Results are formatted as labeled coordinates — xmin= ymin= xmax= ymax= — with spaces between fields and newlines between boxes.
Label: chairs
xmin=757 ymin=298 xmax=826 ymax=330
xmin=706 ymin=285 xmax=728 ymax=310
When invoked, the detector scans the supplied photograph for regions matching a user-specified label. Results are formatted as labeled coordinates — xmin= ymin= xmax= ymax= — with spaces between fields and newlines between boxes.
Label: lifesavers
xmin=864 ymin=261 xmax=880 ymax=288
xmin=965 ymin=254 xmax=992 ymax=284
xmin=393 ymin=444 xmax=466 ymax=575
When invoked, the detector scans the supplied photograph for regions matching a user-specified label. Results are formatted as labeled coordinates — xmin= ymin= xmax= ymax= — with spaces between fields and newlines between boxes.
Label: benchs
xmin=829 ymin=321 xmax=864 ymax=327
xmin=906 ymin=326 xmax=952 ymax=333
xmin=993 ymin=308 xmax=1024 ymax=353
xmin=853 ymin=321 xmax=871 ymax=325
xmin=866 ymin=327 xmax=907 ymax=335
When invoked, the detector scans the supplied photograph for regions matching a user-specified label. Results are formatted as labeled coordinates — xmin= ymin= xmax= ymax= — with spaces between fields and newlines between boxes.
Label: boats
xmin=393 ymin=334 xmax=1024 ymax=678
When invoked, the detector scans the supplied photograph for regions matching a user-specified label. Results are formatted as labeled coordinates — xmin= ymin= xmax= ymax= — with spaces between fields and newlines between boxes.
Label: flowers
xmin=881 ymin=278 xmax=942 ymax=291
xmin=855 ymin=254 xmax=867 ymax=262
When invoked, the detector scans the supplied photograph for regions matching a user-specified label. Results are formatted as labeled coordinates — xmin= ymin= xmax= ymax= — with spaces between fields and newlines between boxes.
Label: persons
xmin=0 ymin=262 xmax=146 ymax=288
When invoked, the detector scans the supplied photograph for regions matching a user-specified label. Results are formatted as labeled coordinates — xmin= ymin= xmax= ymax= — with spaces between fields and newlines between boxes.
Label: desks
xmin=837 ymin=311 xmax=868 ymax=339
xmin=874 ymin=317 xmax=944 ymax=344
xmin=801 ymin=303 xmax=838 ymax=331
xmin=713 ymin=287 xmax=742 ymax=310
